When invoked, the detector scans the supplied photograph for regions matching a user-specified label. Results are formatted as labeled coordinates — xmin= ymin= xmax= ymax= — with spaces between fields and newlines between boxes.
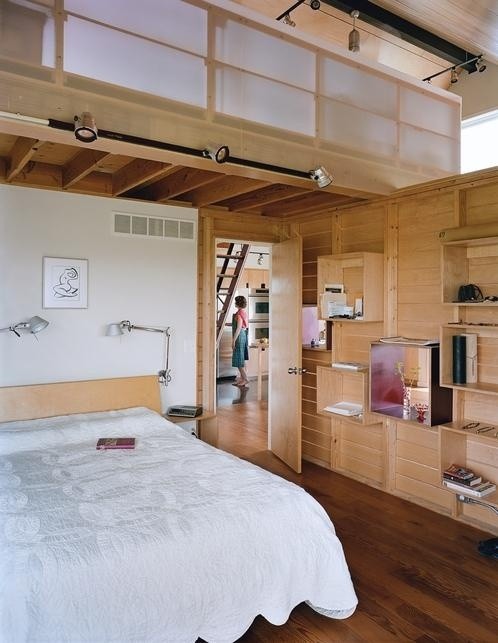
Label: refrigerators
xmin=216 ymin=287 xmax=250 ymax=381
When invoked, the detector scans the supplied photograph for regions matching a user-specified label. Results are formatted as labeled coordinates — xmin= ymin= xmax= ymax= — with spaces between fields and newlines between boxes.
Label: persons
xmin=232 ymin=296 xmax=250 ymax=387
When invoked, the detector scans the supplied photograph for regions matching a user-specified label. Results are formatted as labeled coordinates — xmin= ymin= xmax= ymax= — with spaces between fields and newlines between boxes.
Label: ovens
xmin=249 ymin=288 xmax=269 ymax=347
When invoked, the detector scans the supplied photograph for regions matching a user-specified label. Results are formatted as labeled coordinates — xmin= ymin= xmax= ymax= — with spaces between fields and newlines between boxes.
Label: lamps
xmin=0 ymin=316 xmax=49 ymax=337
xmin=48 ymin=113 xmax=332 ymax=193
xmin=105 ymin=319 xmax=173 ymax=385
xmin=410 ymin=387 xmax=430 ymax=422
xmin=233 ymin=251 xmax=264 ymax=266
xmin=423 ymin=53 xmax=485 ymax=84
xmin=346 ymin=10 xmax=363 ymax=52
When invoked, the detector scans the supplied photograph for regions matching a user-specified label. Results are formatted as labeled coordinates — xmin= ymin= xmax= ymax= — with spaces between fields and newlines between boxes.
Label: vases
xmin=403 ymin=388 xmax=411 ymax=412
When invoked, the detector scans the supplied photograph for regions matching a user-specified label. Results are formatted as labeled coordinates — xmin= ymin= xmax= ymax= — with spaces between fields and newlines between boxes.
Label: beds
xmin=0 ymin=375 xmax=334 ymax=642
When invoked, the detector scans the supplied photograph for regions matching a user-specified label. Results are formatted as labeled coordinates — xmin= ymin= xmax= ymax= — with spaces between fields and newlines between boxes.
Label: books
xmin=443 ymin=464 xmax=497 ymax=498
xmin=332 ymin=362 xmax=368 ymax=369
xmin=96 ymin=438 xmax=135 ymax=449
xmin=324 ymin=402 xmax=362 ymax=416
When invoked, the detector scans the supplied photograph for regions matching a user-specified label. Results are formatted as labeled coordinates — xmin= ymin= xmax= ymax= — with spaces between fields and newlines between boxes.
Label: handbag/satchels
xmin=458 ymin=284 xmax=483 ymax=303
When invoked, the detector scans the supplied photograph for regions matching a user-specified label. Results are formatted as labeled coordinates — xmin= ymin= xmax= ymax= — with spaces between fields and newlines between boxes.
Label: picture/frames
xmin=41 ymin=255 xmax=89 ymax=310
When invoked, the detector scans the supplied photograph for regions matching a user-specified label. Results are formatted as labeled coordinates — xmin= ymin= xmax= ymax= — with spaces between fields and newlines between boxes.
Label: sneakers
xmin=232 ymin=378 xmax=249 ymax=387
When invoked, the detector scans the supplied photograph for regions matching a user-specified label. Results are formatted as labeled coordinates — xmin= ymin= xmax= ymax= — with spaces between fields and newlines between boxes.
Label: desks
xmin=250 ymin=344 xmax=270 ymax=400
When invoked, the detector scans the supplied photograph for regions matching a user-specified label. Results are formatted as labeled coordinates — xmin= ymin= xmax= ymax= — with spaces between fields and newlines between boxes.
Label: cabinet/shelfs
xmin=439 ymin=221 xmax=498 ymax=501
xmin=312 ymin=254 xmax=451 ymax=430
